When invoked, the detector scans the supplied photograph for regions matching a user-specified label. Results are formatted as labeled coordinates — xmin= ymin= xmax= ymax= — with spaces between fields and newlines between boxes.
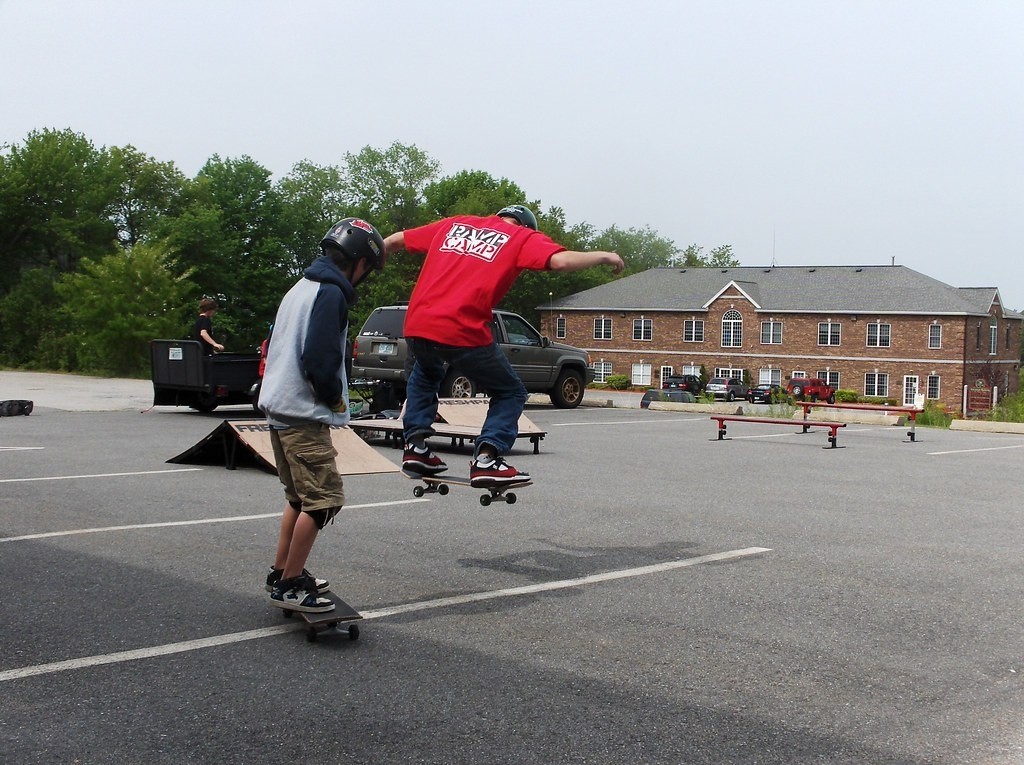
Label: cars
xmin=149 ymin=323 xmax=273 ymax=421
xmin=747 ymin=384 xmax=788 ymax=404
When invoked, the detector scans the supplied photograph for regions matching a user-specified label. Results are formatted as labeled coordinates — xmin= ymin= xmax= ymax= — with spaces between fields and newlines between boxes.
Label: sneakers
xmin=470 ymin=457 xmax=531 ymax=488
xmin=265 ymin=565 xmax=330 ymax=594
xmin=269 ymin=574 xmax=335 ymax=612
xmin=403 ymin=442 xmax=448 ymax=474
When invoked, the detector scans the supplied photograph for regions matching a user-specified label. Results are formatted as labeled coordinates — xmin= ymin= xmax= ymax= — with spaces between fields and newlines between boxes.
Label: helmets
xmin=319 ymin=217 xmax=386 ymax=270
xmin=496 ymin=205 xmax=537 ymax=230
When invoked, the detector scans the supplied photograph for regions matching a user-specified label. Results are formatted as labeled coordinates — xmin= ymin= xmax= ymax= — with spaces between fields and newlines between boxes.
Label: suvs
xmin=662 ymin=375 xmax=706 ymax=398
xmin=787 ymin=378 xmax=836 ymax=406
xmin=350 ymin=305 xmax=596 ymax=409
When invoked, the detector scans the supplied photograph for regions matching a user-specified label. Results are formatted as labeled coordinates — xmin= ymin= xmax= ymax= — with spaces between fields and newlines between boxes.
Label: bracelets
xmin=330 ymin=397 xmax=347 ymax=413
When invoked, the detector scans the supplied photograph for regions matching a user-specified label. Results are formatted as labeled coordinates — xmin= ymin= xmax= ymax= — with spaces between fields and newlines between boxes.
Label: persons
xmin=259 ymin=325 xmax=272 ymax=382
xmin=256 ymin=216 xmax=387 ymax=613
xmin=192 ymin=298 xmax=225 ymax=356
xmin=381 ymin=202 xmax=625 ymax=486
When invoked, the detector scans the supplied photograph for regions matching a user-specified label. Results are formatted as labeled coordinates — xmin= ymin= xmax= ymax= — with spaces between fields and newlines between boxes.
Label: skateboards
xmin=400 ymin=470 xmax=533 ymax=507
xmin=282 ymin=584 xmax=364 ymax=643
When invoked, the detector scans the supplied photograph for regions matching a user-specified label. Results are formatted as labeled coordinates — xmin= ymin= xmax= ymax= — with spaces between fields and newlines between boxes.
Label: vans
xmin=705 ymin=377 xmax=751 ymax=402
